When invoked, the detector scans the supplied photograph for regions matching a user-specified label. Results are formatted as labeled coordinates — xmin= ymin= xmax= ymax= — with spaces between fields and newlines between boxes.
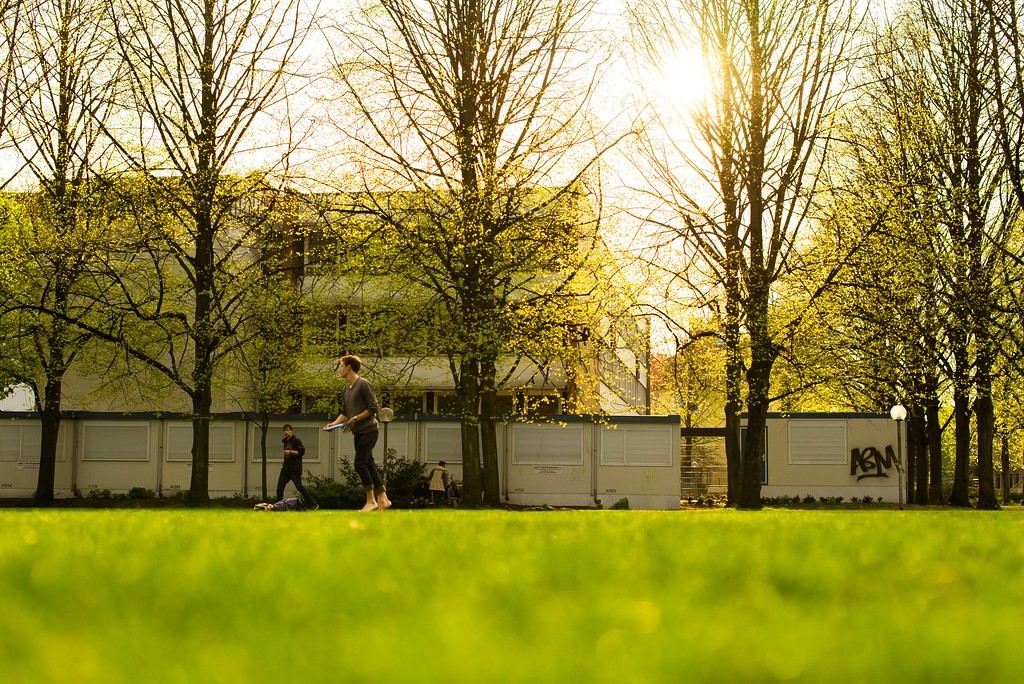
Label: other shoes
xmin=410 ymin=502 xmax=415 ymax=504
xmin=313 ymin=503 xmax=319 ymax=510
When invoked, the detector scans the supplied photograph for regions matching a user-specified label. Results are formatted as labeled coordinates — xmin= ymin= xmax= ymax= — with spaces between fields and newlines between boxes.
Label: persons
xmin=429 ymin=461 xmax=449 ymax=508
xmin=276 ymin=424 xmax=319 ymax=510
xmin=323 ymin=356 xmax=392 ymax=510
xmin=413 ymin=476 xmax=432 ymax=509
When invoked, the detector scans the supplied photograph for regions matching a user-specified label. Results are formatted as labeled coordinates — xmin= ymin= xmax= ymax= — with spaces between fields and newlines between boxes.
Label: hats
xmin=439 ymin=461 xmax=447 ymax=465
xmin=418 ymin=476 xmax=426 ymax=480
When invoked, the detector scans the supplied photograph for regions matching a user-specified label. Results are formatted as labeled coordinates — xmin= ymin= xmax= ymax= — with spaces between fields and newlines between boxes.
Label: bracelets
xmin=290 ymin=450 xmax=291 ymax=454
xmin=354 ymin=415 xmax=358 ymax=422
